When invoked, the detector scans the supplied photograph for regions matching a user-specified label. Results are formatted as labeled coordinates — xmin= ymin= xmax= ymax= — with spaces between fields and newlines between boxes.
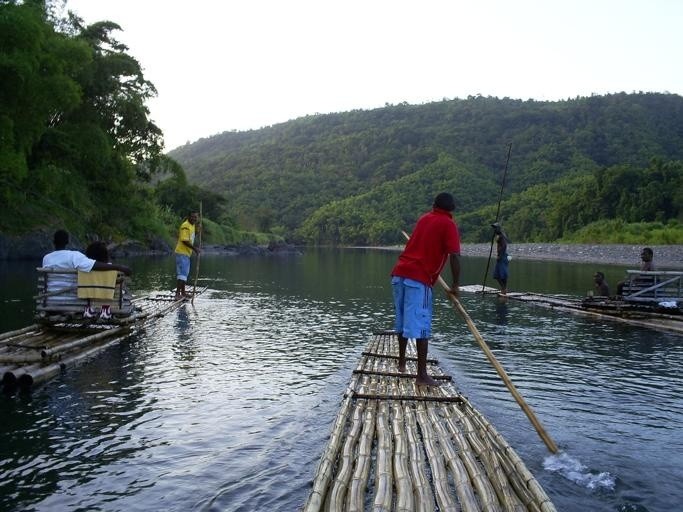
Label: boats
xmin=458 ymin=268 xmax=682 ymax=337
xmin=303 ymin=327 xmax=560 ymax=511
xmin=0 ymin=283 xmax=208 ymax=389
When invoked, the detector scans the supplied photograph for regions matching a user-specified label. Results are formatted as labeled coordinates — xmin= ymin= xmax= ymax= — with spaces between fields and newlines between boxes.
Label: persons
xmin=80 ymin=242 xmax=130 ymax=310
xmin=614 ymin=247 xmax=661 ymax=296
xmin=171 ymin=210 xmax=200 ymax=300
xmin=388 ymin=193 xmax=461 ymax=387
xmin=592 ymin=271 xmax=608 ymax=296
xmin=35 ymin=229 xmax=131 ymax=322
xmin=490 ymin=223 xmax=509 ymax=295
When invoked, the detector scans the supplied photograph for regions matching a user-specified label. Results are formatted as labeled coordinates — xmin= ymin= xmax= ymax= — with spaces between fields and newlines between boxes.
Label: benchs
xmin=33 ymin=264 xmax=149 ymax=331
xmin=582 ymin=270 xmax=683 ymax=317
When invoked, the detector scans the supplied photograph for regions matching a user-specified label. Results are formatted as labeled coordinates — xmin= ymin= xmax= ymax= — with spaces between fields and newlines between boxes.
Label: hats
xmin=490 ymin=222 xmax=501 ymax=228
